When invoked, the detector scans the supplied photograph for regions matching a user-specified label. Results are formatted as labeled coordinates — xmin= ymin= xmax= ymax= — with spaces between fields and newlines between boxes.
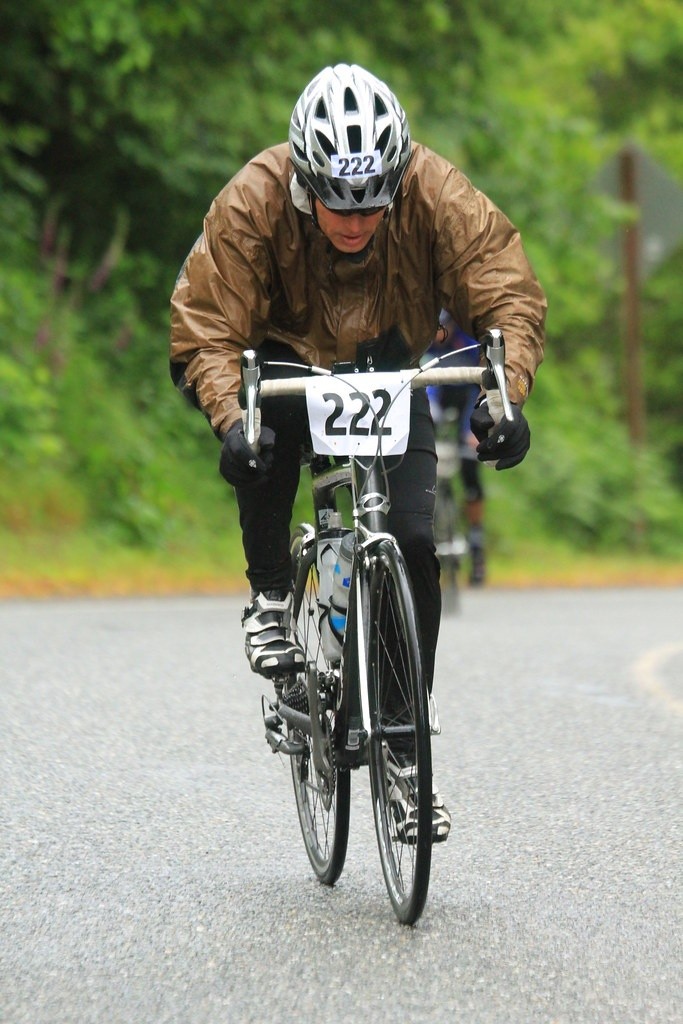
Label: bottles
xmin=316 ymin=512 xmax=366 ymax=662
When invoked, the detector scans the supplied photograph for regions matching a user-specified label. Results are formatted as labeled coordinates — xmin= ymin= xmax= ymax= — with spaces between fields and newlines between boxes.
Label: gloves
xmin=470 ymin=400 xmax=531 ymax=472
xmin=219 ymin=422 xmax=275 ymax=487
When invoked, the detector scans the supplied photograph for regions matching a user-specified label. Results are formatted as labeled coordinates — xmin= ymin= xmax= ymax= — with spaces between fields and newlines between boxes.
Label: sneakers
xmin=385 ymin=747 xmax=450 ymax=844
xmin=240 ymin=593 xmax=307 ymax=675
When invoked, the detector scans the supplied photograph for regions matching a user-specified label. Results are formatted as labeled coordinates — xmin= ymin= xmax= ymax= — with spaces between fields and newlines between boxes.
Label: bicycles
xmin=241 ymin=326 xmax=517 ymax=926
xmin=429 ymin=412 xmax=471 ymax=619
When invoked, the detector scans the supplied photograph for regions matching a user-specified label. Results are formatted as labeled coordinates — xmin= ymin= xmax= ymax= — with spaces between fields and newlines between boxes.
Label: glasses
xmin=330 ymin=206 xmax=385 ymax=216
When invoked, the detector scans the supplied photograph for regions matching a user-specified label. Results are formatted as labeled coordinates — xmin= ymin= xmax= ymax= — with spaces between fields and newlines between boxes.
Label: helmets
xmin=286 ymin=64 xmax=410 ymax=210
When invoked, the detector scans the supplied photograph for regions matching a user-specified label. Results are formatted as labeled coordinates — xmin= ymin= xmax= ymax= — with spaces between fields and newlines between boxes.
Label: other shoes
xmin=468 ymin=557 xmax=484 ymax=584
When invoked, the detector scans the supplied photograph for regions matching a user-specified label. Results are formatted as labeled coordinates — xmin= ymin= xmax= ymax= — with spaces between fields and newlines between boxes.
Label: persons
xmin=420 ymin=307 xmax=487 ymax=585
xmin=169 ymin=60 xmax=546 ymax=840
